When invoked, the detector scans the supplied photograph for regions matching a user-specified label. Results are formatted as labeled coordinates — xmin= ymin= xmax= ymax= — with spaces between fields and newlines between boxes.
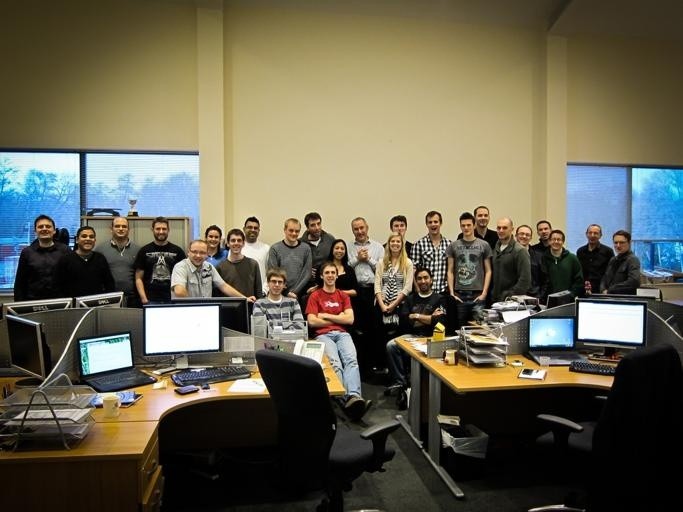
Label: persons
xmin=250 ymin=267 xmax=303 ymax=338
xmin=130 ymin=216 xmax=187 ymax=304
xmin=93 ymin=215 xmax=142 ymax=308
xmin=198 ymin=204 xmax=643 ymax=321
xmin=12 ymin=213 xmax=84 ymax=303
xmin=380 ymin=267 xmax=449 ymax=410
xmin=303 ymin=262 xmax=373 ymax=421
xmin=169 ymin=239 xmax=256 ymax=305
xmin=69 ymin=225 xmax=115 ymax=295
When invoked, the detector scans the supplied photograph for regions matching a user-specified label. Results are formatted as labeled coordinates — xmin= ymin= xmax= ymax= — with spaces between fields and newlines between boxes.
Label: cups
xmin=102 ymin=395 xmax=120 ymax=418
xmin=540 ymin=356 xmax=549 ymax=374
xmin=441 ymin=349 xmax=458 ymax=365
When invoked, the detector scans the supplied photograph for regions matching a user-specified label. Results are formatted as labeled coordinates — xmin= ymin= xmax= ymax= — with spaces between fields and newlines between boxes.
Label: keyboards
xmin=569 ymin=361 xmax=617 ymax=375
xmin=171 ymin=365 xmax=251 ymax=387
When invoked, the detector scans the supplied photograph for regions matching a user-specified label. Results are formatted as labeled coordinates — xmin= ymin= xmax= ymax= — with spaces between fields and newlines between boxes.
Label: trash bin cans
xmin=440 ymin=423 xmax=489 ymax=482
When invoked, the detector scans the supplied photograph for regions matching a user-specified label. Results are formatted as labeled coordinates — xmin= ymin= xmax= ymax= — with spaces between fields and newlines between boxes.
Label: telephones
xmin=293 ymin=339 xmax=325 ymax=368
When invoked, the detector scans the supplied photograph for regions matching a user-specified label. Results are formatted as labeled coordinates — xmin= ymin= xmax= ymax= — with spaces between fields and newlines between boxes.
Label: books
xmin=95 ymin=393 xmax=142 ymax=408
xmin=89 ymin=390 xmax=135 ymax=406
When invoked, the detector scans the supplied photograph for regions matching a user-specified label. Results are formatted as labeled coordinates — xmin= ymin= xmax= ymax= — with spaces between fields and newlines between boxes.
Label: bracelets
xmin=324 ymin=312 xmax=328 ymax=319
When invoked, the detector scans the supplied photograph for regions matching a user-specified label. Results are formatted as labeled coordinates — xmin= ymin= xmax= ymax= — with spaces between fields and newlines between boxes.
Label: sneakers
xmin=351 ymin=400 xmax=371 ymax=422
xmin=395 ymin=393 xmax=407 ymax=411
xmin=384 ymin=384 xmax=405 ymax=396
xmin=345 ymin=397 xmax=365 ymax=415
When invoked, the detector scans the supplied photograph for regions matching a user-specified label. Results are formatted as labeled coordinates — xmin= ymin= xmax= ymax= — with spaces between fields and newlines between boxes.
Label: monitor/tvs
xmin=636 ymin=288 xmax=662 ymax=302
xmin=546 ymin=290 xmax=572 ymax=310
xmin=3 ymin=297 xmax=73 ymax=320
xmin=6 ymin=315 xmax=55 ymax=389
xmin=73 ymin=291 xmax=124 ymax=309
xmin=172 ymin=297 xmax=250 ymax=334
xmin=575 ymin=298 xmax=648 ymax=363
xmin=142 ymin=304 xmax=222 ymax=376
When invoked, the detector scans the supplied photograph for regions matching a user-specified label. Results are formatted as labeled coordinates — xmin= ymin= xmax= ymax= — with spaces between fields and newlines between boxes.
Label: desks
xmin=0 ymin=360 xmax=347 ymax=424
xmin=395 ymin=335 xmax=453 ymax=450
xmin=426 ymin=350 xmax=611 ymax=501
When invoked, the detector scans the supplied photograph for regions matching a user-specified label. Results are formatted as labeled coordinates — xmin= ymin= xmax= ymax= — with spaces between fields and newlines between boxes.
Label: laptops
xmin=77 ymin=330 xmax=157 ymax=393
xmin=527 ymin=316 xmax=589 ymax=366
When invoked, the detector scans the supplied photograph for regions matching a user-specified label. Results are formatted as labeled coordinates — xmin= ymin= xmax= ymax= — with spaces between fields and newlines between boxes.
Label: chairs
xmin=250 ymin=348 xmax=401 ymax=512
xmin=525 ymin=340 xmax=682 ymax=512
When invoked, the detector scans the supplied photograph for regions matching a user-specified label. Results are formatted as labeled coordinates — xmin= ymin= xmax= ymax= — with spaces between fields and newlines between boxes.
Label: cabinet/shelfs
xmin=0 ymin=421 xmax=163 ymax=512
xmin=80 ymin=215 xmax=193 ymax=259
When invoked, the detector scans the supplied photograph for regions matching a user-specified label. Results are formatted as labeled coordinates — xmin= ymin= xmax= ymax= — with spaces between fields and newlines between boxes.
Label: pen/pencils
xmin=2 ymin=384 xmax=10 ymax=399
